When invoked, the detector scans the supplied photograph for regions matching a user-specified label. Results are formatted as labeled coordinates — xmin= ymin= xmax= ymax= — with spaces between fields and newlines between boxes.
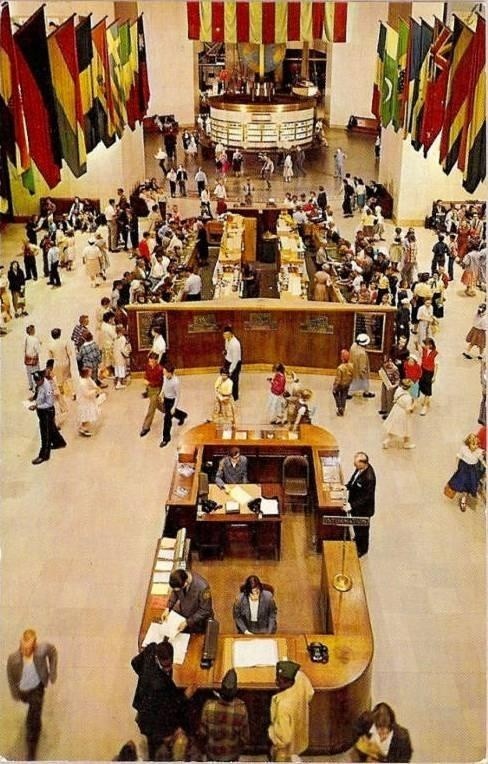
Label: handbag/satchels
xmin=444 ymin=482 xmax=458 ymax=499
xmin=17 ymin=292 xmax=25 ymax=305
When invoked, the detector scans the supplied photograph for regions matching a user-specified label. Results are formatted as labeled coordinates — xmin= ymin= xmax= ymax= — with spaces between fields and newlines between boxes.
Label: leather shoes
xmin=32 ymin=457 xmax=50 ymax=464
xmin=140 ymin=428 xmax=150 ymax=437
xmin=178 ymin=413 xmax=187 ymax=425
xmin=51 ymin=442 xmax=66 ymax=449
xmin=357 ymin=551 xmax=367 ymax=557
xmin=159 ymin=440 xmax=170 ymax=447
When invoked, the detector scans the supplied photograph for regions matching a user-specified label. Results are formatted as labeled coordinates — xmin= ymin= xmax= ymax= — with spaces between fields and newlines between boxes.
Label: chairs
xmin=281 ymin=455 xmax=311 ymax=513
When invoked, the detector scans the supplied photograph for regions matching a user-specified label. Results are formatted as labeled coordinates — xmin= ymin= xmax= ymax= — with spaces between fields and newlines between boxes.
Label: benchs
xmin=368 ymin=181 xmax=393 ymax=218
xmin=431 ymin=202 xmax=487 ymax=228
xmin=40 ymin=197 xmax=100 ymax=225
xmin=350 ymin=115 xmax=380 ymax=136
xmin=142 ymin=114 xmax=179 ymax=134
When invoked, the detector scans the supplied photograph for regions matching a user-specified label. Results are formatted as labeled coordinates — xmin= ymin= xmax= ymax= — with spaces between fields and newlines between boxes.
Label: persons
xmin=309 ymin=205 xmax=338 ymax=238
xmin=448 ymin=234 xmax=458 ymax=280
xmin=332 ymin=349 xmax=353 ymax=416
xmin=94 ymin=232 xmax=110 ymax=280
xmin=289 ymin=385 xmax=303 ymax=423
xmin=41 ymin=368 xmax=63 ymax=429
xmin=463 ymin=304 xmax=487 ymax=359
xmin=438 ymin=265 xmax=448 ymax=288
xmin=233 ymin=575 xmax=277 ymax=635
xmin=132 ymin=641 xmax=192 ymax=762
xmin=261 ymin=156 xmax=274 ymax=190
xmin=38 ymin=196 xmax=56 ymax=227
xmin=195 ymin=165 xmax=209 ymax=195
xmin=212 ymin=367 xmax=236 ymax=423
xmin=316 ymin=239 xmax=334 ymax=270
xmin=8 ymin=260 xmax=28 ymax=318
xmin=351 ymin=701 xmax=412 ymax=762
xmin=416 ymin=296 xmax=434 ymax=344
xmin=314 ymin=262 xmax=333 ymax=299
xmin=478 ymin=361 xmax=488 ymax=425
xmin=68 ymin=196 xmax=85 ymax=229
xmin=267 ymin=660 xmax=314 ymax=761
xmin=222 ymin=325 xmax=243 ymax=401
xmin=24 ymin=325 xmax=40 ymax=389
xmin=336 ymin=265 xmax=398 ymax=304
xmin=110 ymin=310 xmax=120 ymax=340
xmin=308 ymin=191 xmax=318 ymax=204
xmin=219 ymin=148 xmax=228 ymax=177
xmin=0 ymin=265 xmax=12 ymax=322
xmin=411 ymin=271 xmax=432 ymax=335
xmin=156 ymin=729 xmax=201 ymax=762
xmin=477 ymin=426 xmax=488 ymax=449
xmin=202 ymin=210 xmax=212 ymax=217
xmin=283 ymin=153 xmax=294 ymax=181
xmin=346 ymin=114 xmax=357 ymax=131
xmin=142 ymin=325 xmax=168 ymax=397
xmin=317 ymin=185 xmax=327 ymax=207
xmin=379 ymin=379 xmax=416 ymax=448
xmin=101 ymin=313 xmax=113 ymax=378
xmin=378 ymin=358 xmax=401 ymax=418
xmin=375 ymin=135 xmax=380 ymax=158
xmin=109 ymin=324 xmax=129 ymax=389
xmin=123 ymin=250 xmax=179 ymax=304
xmin=106 ymin=187 xmax=138 ymax=252
xmin=415 ymin=338 xmax=439 ymax=415
xmin=74 ymin=367 xmax=101 ymax=436
xmin=62 ymin=213 xmax=74 ymax=236
xmin=399 ymin=278 xmax=413 ymax=307
xmin=167 ymin=167 xmax=177 ymax=197
xmin=342 ymin=452 xmax=376 ymax=558
xmin=448 ymin=433 xmax=487 ymax=512
xmin=63 ymin=228 xmax=75 ymax=270
xmin=72 ymin=314 xmax=107 ymax=387
xmin=293 ymin=205 xmax=307 ymax=242
xmin=445 ymin=205 xmax=458 ymax=234
xmin=96 ymin=296 xmax=114 ymax=354
xmin=411 ymin=272 xmax=423 ymax=291
xmin=183 ymin=129 xmax=199 ymax=156
xmin=201 ymin=184 xmax=213 ymax=218
xmin=196 ymin=222 xmax=210 ymax=267
xmin=242 ymin=176 xmax=256 ymax=204
xmin=431 ymin=234 xmax=449 ymax=275
xmin=339 ymin=173 xmax=383 ymax=217
xmin=82 ymin=238 xmax=103 ymax=287
xmin=0 ymin=296 xmax=8 ymax=333
xmin=29 ymin=371 xmax=66 ymax=464
xmin=114 ymin=297 xmax=128 ymax=332
xmin=292 ymin=389 xmax=312 ymax=433
xmin=79 ymin=331 xmax=99 ymax=382
xmin=177 ymin=164 xmax=185 ymax=173
xmin=159 ymin=361 xmax=187 ymax=448
xmin=83 ymin=198 xmax=97 ymax=231
xmin=290 ymin=371 xmax=301 ymax=393
xmin=214 ymin=446 xmax=248 ymax=494
xmin=140 ymin=352 xmax=166 ymax=436
xmin=40 ymin=234 xmax=52 ymax=276
xmin=112 ymin=279 xmax=122 ymax=307
xmin=46 ymin=357 xmax=69 ymax=413
xmin=389 ymin=227 xmax=420 ymax=272
xmin=284 ymin=192 xmax=292 ymax=202
xmin=334 ymin=147 xmax=346 ymax=176
xmin=348 ymin=333 xmax=376 ymax=398
xmin=242 ymin=264 xmax=261 ymax=297
xmin=161 ymin=568 xmax=214 ymax=633
xmin=7 ymin=629 xmax=57 ymax=760
xmin=139 ymin=177 xmax=166 ymax=204
xmin=215 ymin=140 xmax=224 ymax=173
xmin=338 ymin=239 xmax=386 ymax=264
xmin=429 ymin=270 xmax=445 ymax=318
xmin=26 ymin=214 xmax=38 ymax=244
xmin=294 ymin=145 xmax=307 ymax=177
xmin=266 ymin=362 xmax=286 ymax=424
xmin=395 ymin=299 xmax=410 ymax=350
xmin=95 ymin=213 xmax=109 ymax=250
xmin=23 ymin=238 xmax=38 ymax=280
xmin=154 ymin=113 xmax=178 ymax=131
xmin=314 ymin=117 xmax=328 ymax=146
xmin=287 ymin=197 xmax=291 ymax=202
xmin=356 ymin=205 xmax=385 ymax=239
xmin=402 ymin=354 xmax=423 ymax=413
xmin=56 ymin=222 xmax=67 ymax=246
xmin=46 ymin=240 xmax=61 ymax=286
xmin=177 ymin=165 xmax=188 ymax=196
xmin=141 ymin=204 xmax=194 ymax=250
xmin=458 ymin=203 xmax=487 ymax=296
xmin=214 ymin=178 xmax=228 ymax=214
xmin=292 ymin=194 xmax=297 ymax=203
xmin=300 ymin=192 xmax=306 ymax=201
xmin=183 ymin=266 xmax=203 ymax=300
xmin=431 ymin=199 xmax=447 ymax=234
xmin=384 ymin=335 xmax=409 ymax=380
xmin=112 ymin=739 xmax=141 ymax=761
xmin=232 ymin=148 xmax=244 ymax=176
xmin=48 ymin=328 xmax=80 ymax=393
xmin=199 ymin=668 xmax=252 ymax=762
xmin=197 ymin=216 xmax=202 ymax=223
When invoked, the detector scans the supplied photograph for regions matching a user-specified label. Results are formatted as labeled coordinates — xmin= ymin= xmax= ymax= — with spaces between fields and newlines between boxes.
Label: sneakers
xmin=95 ymin=283 xmax=100 ymax=287
xmin=15 ymin=312 xmax=19 ymax=318
xmin=363 ymin=390 xmax=375 ymax=398
xmin=112 ymin=249 xmax=120 ymax=252
xmin=403 ymin=443 xmax=415 ymax=449
xmin=420 ymin=407 xmax=427 ymax=416
xmin=382 ymin=441 xmax=389 ymax=449
xmin=480 ymin=289 xmax=485 ymax=292
xmin=109 ymin=247 xmax=112 ymax=251
xmin=460 ymin=499 xmax=467 ymax=512
xmin=47 ymin=282 xmax=55 ymax=285
xmin=476 ymin=284 xmax=482 ymax=288
xmin=22 ymin=311 xmax=28 ymax=316
xmin=378 ymin=410 xmax=387 ymax=415
xmin=54 ymin=283 xmax=61 ymax=286
xmin=45 ymin=273 xmax=49 ymax=277
xmin=382 ymin=415 xmax=389 ymax=419
xmin=99 ymin=383 xmax=108 ymax=388
xmin=116 ymin=384 xmax=128 ymax=390
xmin=25 ymin=277 xmax=32 ymax=280
xmin=411 ymin=329 xmax=418 ymax=334
xmin=478 ymin=354 xmax=485 ymax=359
xmin=338 ymin=407 xmax=343 ymax=415
xmin=463 ymin=351 xmax=473 ymax=360
xmin=34 ymin=277 xmax=37 ymax=281
xmin=346 ymin=395 xmax=352 ymax=400
xmin=79 ymin=428 xmax=91 ymax=436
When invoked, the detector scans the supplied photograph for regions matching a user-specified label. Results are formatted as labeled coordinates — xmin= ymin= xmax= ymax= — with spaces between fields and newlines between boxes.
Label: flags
xmin=372 ymin=22 xmax=399 ymax=128
xmin=48 ymin=17 xmax=87 ymax=178
xmin=401 ymin=18 xmax=421 ymax=139
xmin=0 ymin=2 xmax=35 ymax=221
xmin=12 ymin=7 xmax=64 ymax=190
xmin=409 ymin=20 xmax=433 ymax=151
xmin=137 ymin=15 xmax=150 ymax=109
xmin=439 ymin=14 xmax=473 ymax=176
xmin=416 ymin=17 xmax=454 ymax=158
xmin=186 ymin=0 xmax=347 ymax=43
xmin=91 ymin=18 xmax=116 ymax=147
xmin=106 ymin=22 xmax=128 ymax=140
xmin=75 ymin=16 xmax=102 ymax=153
xmin=459 ymin=16 xmax=488 ymax=194
xmin=130 ymin=20 xmax=146 ymax=124
xmin=392 ymin=19 xmax=410 ymax=133
xmin=120 ymin=22 xmax=136 ymax=131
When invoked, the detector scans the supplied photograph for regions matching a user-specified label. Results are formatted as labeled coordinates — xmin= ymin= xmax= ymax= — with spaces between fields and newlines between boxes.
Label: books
xmin=151 ymin=583 xmax=168 ymax=594
xmin=184 ymin=538 xmax=190 ymax=560
xmin=229 ymin=485 xmax=252 ymax=504
xmin=151 ymin=571 xmax=171 ymax=581
xmin=330 ymin=490 xmax=349 ymax=499
xmin=151 ymin=595 xmax=168 ymax=610
xmin=158 ymin=536 xmax=176 ymax=548
xmin=155 ymin=560 xmax=173 ymax=570
xmin=259 ymin=497 xmax=279 ymax=514
xmin=161 ymin=610 xmax=187 ymax=638
xmin=233 ymin=638 xmax=278 ymax=667
xmin=225 ymin=501 xmax=239 ymax=509
xmin=141 ymin=621 xmax=190 ymax=665
xmin=174 ymin=527 xmax=186 ymax=559
xmin=157 ymin=548 xmax=175 ymax=560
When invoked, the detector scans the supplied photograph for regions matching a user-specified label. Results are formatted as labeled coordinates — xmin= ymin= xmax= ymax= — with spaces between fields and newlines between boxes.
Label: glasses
xmin=232 ymin=455 xmax=240 ymax=459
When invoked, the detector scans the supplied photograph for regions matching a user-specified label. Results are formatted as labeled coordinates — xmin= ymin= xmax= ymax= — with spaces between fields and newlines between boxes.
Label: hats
xmin=221 ymin=667 xmax=238 ymax=696
xmin=32 ymin=370 xmax=46 ymax=379
xmin=276 ymin=660 xmax=300 ymax=679
xmin=355 ymin=332 xmax=370 ymax=346
